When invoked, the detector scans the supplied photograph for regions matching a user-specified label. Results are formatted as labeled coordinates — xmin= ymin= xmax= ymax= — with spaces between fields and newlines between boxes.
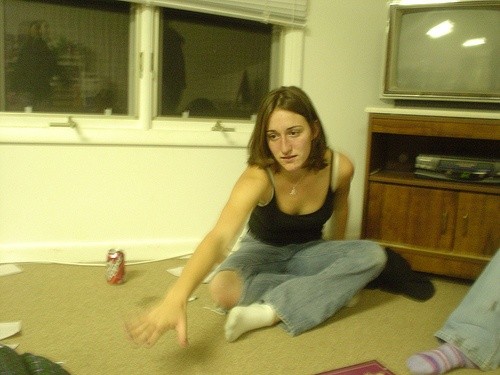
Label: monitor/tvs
xmin=379 ymin=0 xmax=500 ymax=103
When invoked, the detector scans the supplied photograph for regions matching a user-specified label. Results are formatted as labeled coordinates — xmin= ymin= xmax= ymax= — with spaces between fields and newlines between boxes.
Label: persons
xmin=125 ymin=85 xmax=387 ymax=349
xmin=405 ymin=249 xmax=500 ymax=375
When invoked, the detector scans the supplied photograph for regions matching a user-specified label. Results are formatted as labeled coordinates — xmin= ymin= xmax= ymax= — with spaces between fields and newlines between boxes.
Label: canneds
xmin=106 ymin=249 xmax=126 ymax=286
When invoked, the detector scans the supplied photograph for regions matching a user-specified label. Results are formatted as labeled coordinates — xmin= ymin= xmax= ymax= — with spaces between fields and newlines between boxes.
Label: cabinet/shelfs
xmin=361 ymin=112 xmax=500 ymax=280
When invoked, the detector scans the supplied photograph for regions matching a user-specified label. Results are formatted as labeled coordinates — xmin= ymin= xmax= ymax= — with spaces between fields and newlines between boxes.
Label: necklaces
xmin=290 ymin=171 xmax=307 ymax=195
